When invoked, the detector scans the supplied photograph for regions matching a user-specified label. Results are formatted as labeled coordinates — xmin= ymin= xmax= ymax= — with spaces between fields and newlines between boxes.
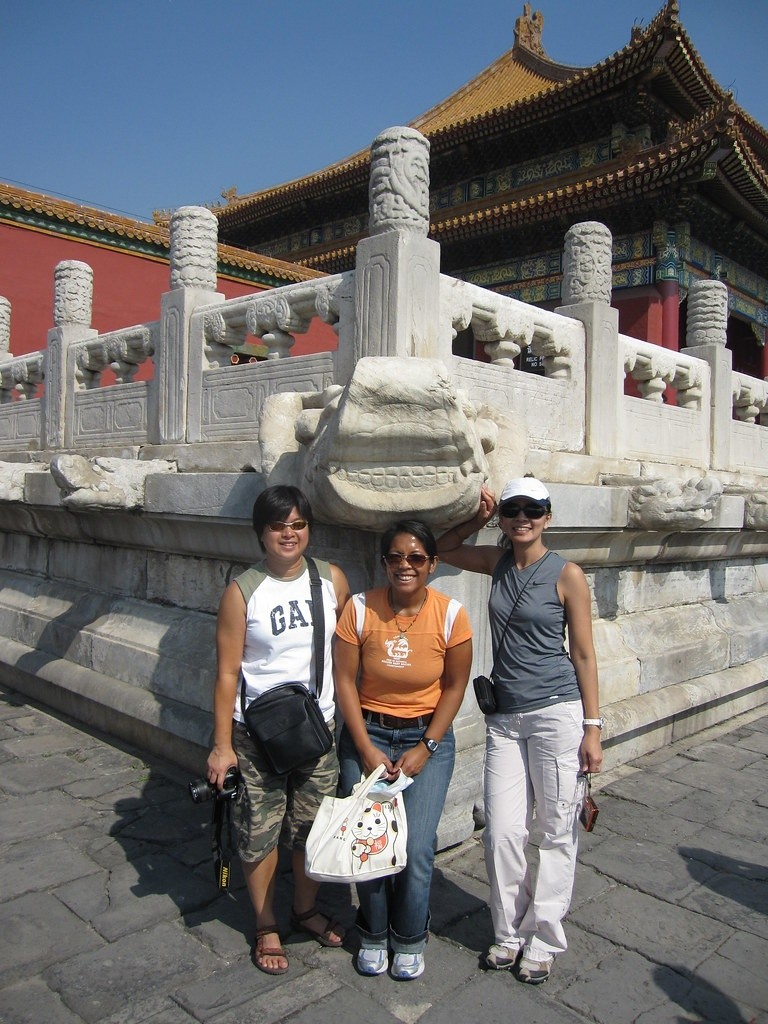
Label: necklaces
xmin=391 ymin=587 xmax=427 ymax=639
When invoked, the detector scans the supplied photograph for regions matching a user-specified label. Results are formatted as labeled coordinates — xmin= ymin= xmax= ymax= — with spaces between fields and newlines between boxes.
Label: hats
xmin=499 ymin=477 xmax=551 ymax=505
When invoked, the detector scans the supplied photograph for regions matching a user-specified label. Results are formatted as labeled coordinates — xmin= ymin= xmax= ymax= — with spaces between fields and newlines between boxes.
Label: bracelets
xmin=436 ymin=477 xmax=603 ymax=983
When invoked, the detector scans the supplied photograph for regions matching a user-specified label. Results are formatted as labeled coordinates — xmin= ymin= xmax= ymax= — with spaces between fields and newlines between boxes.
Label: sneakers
xmin=391 ymin=953 xmax=425 ymax=979
xmin=357 ymin=949 xmax=389 ymax=974
xmin=485 ymin=944 xmax=519 ymax=968
xmin=518 ymin=953 xmax=559 ymax=984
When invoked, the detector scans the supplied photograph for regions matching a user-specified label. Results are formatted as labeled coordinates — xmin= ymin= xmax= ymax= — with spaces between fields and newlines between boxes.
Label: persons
xmin=205 ymin=486 xmax=351 ymax=974
xmin=332 ymin=521 xmax=473 ymax=980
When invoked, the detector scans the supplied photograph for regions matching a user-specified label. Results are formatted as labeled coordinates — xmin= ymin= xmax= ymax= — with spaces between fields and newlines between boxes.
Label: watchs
xmin=421 ymin=737 xmax=439 ymax=753
xmin=584 ymin=719 xmax=603 ymax=730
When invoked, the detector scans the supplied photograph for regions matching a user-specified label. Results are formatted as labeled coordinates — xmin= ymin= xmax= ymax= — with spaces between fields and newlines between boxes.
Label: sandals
xmin=290 ymin=907 xmax=349 ymax=946
xmin=254 ymin=922 xmax=291 ymax=974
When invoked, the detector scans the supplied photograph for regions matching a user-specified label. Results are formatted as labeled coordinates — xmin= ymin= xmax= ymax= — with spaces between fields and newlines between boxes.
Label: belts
xmin=361 ymin=709 xmax=435 ymax=730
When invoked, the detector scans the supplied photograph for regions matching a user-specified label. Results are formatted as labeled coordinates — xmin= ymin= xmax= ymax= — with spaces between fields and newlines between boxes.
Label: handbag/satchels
xmin=245 ymin=682 xmax=333 ymax=775
xmin=472 ymin=676 xmax=498 ymax=714
xmin=305 ymin=763 xmax=414 ymax=883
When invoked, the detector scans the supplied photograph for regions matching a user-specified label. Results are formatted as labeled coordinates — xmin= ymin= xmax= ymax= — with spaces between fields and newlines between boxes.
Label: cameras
xmin=579 ymin=796 xmax=600 ymax=832
xmin=187 ymin=768 xmax=239 ymax=804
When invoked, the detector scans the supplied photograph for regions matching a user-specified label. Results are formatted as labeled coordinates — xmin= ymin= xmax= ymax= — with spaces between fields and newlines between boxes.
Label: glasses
xmin=264 ymin=519 xmax=309 ymax=531
xmin=500 ymin=502 xmax=548 ymax=519
xmin=384 ymin=554 xmax=433 ymax=567
